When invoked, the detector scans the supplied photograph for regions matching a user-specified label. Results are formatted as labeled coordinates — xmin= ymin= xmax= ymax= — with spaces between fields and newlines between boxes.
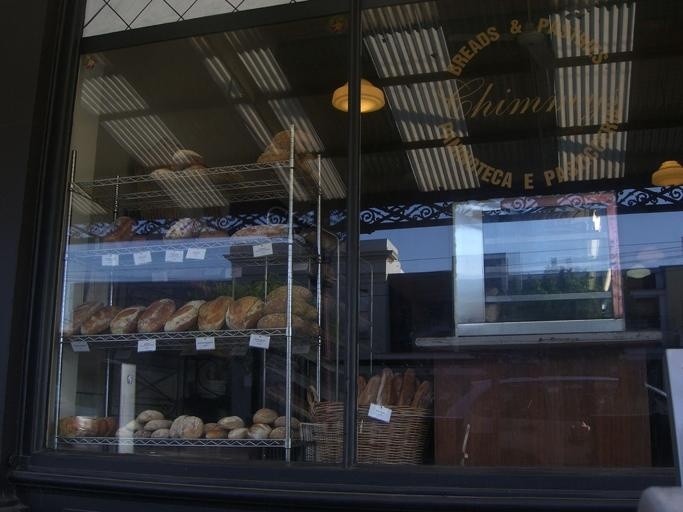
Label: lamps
xmin=626 ymin=268 xmax=652 ymax=279
xmin=331 ymin=78 xmax=387 ymax=114
xmin=651 ymin=160 xmax=683 ymax=187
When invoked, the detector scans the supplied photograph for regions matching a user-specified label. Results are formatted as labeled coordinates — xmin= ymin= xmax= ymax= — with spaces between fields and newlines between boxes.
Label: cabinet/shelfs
xmin=51 ymin=124 xmax=324 ymax=464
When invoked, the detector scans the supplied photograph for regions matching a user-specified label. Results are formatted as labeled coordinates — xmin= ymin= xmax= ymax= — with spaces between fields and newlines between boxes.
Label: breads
xmin=166 ymin=217 xmax=195 ymax=240
xmin=274 ymin=130 xmax=291 ymax=148
xmin=62 ymin=285 xmax=317 ymax=335
xmin=358 ymin=365 xmax=434 ymax=408
xmin=256 ymin=150 xmax=291 ymax=162
xmin=150 ymin=149 xmax=207 ymax=174
xmin=103 ymin=216 xmax=138 ymax=242
xmin=232 ymin=224 xmax=288 ymax=237
xmin=58 ymin=408 xmax=300 ymax=439
xmin=197 ymin=230 xmax=229 ymax=238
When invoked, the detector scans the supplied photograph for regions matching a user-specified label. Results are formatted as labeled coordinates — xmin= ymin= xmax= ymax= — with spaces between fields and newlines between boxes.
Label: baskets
xmin=305 ymin=385 xmax=433 ymax=466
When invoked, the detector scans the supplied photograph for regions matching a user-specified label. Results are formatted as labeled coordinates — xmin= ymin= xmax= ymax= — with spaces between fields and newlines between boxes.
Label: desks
xmin=414 ymin=329 xmax=664 ymax=470
